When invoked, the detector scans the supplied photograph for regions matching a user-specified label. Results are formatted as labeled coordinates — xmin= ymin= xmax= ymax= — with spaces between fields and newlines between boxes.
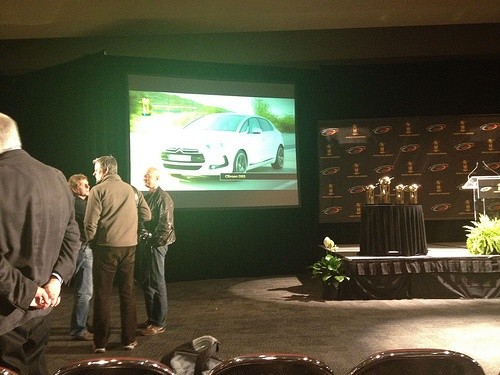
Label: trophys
xmin=393 ymin=184 xmax=408 ymax=205
xmin=407 ymin=183 xmax=421 ymax=204
xmin=362 ymin=184 xmax=378 ymax=205
xmin=376 ymin=176 xmax=395 ymax=205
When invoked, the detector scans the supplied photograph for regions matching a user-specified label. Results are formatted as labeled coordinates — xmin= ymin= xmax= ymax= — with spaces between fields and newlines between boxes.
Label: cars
xmin=158 ymin=112 xmax=286 ymax=182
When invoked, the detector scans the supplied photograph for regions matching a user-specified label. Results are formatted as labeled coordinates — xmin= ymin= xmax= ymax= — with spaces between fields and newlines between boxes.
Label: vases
xmin=321 ymin=280 xmax=340 ymax=300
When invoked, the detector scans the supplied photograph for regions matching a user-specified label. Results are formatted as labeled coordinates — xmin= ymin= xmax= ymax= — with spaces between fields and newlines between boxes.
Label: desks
xmin=357 ymin=204 xmax=429 ymax=256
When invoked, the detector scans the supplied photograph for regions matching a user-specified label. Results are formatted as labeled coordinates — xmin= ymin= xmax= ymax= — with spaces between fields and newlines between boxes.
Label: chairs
xmin=209 ymin=352 xmax=336 ymax=375
xmin=346 ymin=349 xmax=486 ymax=375
xmin=50 ymin=356 xmax=175 ymax=375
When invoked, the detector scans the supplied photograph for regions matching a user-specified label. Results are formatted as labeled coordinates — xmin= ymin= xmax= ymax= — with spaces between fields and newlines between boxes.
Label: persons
xmin=0 ymin=114 xmax=82 ymax=375
xmin=136 ymin=168 xmax=176 ymax=335
xmin=69 ymin=174 xmax=94 ymax=341
xmin=82 ymin=156 xmax=152 ymax=353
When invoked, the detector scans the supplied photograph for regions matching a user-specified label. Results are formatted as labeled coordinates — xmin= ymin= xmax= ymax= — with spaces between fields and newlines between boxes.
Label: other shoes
xmin=71 ymin=331 xmax=94 ymax=340
xmin=94 ymin=344 xmax=106 ymax=353
xmin=123 ymin=341 xmax=138 ymax=349
xmin=137 ymin=322 xmax=165 ymax=335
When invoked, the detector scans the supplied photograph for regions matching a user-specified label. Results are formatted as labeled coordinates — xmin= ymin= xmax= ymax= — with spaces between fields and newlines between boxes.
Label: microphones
xmin=482 ymin=160 xmax=500 ymax=176
xmin=467 ymin=161 xmax=479 ymax=180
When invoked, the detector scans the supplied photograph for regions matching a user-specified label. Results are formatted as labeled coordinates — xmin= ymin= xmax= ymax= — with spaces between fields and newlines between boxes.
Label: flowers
xmin=461 ymin=211 xmax=500 ymax=256
xmin=305 ymin=236 xmax=351 ymax=282
